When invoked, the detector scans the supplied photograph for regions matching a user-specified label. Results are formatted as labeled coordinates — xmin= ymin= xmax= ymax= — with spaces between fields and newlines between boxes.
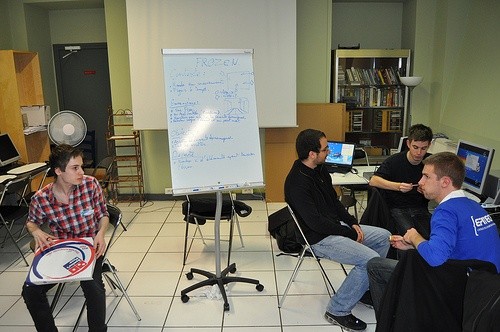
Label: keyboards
xmin=7 ymin=162 xmax=47 ymax=174
xmin=0 ymin=174 xmax=16 ymax=184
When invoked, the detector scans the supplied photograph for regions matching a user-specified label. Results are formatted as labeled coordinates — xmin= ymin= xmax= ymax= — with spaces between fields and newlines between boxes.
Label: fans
xmin=48 ymin=110 xmax=87 ymax=147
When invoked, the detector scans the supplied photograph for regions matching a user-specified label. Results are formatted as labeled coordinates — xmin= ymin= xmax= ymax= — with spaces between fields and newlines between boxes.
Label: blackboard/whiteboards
xmin=161 ymin=49 xmax=265 ymax=196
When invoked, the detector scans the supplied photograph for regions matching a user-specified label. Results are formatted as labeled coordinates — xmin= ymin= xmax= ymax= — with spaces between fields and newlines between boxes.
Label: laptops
xmin=323 ymin=140 xmax=355 ymax=174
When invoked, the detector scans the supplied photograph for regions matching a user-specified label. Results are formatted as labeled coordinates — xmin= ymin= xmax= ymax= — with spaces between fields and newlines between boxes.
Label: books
xmin=337 ymin=65 xmax=406 ymax=107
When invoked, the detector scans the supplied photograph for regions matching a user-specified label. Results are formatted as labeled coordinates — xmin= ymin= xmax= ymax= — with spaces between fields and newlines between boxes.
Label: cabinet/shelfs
xmin=0 ymin=49 xmax=52 ymax=194
xmin=333 ymin=49 xmax=412 ymax=155
xmin=105 ymin=108 xmax=146 ymax=207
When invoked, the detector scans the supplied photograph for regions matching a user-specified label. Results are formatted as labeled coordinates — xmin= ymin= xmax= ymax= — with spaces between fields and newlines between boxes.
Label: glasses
xmin=320 ymin=149 xmax=330 ymax=154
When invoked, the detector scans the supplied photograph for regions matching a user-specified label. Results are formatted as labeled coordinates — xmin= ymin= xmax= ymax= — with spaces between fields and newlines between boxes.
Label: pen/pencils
xmin=412 ymin=185 xmax=419 ymax=186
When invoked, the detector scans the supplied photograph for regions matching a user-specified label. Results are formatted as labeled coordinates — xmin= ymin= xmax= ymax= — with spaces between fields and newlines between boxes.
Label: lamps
xmin=399 ymin=76 xmax=424 ymax=136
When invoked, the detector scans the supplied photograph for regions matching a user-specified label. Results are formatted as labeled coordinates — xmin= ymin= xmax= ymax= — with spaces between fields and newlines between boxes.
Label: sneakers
xmin=323 ymin=310 xmax=367 ymax=332
xmin=357 ymin=290 xmax=374 ymax=309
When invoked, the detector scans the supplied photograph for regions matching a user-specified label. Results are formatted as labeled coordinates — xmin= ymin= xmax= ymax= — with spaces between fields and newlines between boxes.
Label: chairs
xmin=79 ymin=131 xmax=95 ymax=169
xmin=0 ymin=164 xmax=51 ymax=266
xmin=92 ymin=155 xmax=127 ymax=231
xmin=48 ymin=202 xmax=142 ymax=332
xmin=279 ymin=201 xmax=348 ymax=307
xmin=181 ymin=191 xmax=243 ymax=265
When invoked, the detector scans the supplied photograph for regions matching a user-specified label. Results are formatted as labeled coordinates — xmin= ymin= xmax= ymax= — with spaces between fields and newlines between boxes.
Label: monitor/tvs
xmin=0 ymin=133 xmax=22 ymax=166
xmin=455 ymin=139 xmax=495 ymax=194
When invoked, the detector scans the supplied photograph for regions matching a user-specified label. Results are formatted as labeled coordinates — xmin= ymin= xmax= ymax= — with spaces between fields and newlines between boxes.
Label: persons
xmin=367 ymin=152 xmax=496 ymax=320
xmin=368 ymin=123 xmax=432 ymax=261
xmin=284 ymin=129 xmax=392 ymax=332
xmin=21 ymin=143 xmax=110 ymax=332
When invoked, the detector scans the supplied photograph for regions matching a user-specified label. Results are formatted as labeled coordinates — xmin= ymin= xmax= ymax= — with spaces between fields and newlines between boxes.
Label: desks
xmin=0 ymin=163 xmax=49 ymax=194
xmin=353 ymin=155 xmax=389 ymax=165
xmin=330 ymin=171 xmax=370 ymax=216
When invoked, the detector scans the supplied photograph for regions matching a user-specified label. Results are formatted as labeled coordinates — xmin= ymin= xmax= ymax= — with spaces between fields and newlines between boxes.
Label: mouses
xmin=350 ymin=168 xmax=358 ymax=174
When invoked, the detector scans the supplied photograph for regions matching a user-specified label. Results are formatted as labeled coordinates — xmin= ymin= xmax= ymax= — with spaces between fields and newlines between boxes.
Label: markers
xmin=219 ymin=186 xmax=231 ymax=189
xmin=193 ymin=188 xmax=213 ymax=191
